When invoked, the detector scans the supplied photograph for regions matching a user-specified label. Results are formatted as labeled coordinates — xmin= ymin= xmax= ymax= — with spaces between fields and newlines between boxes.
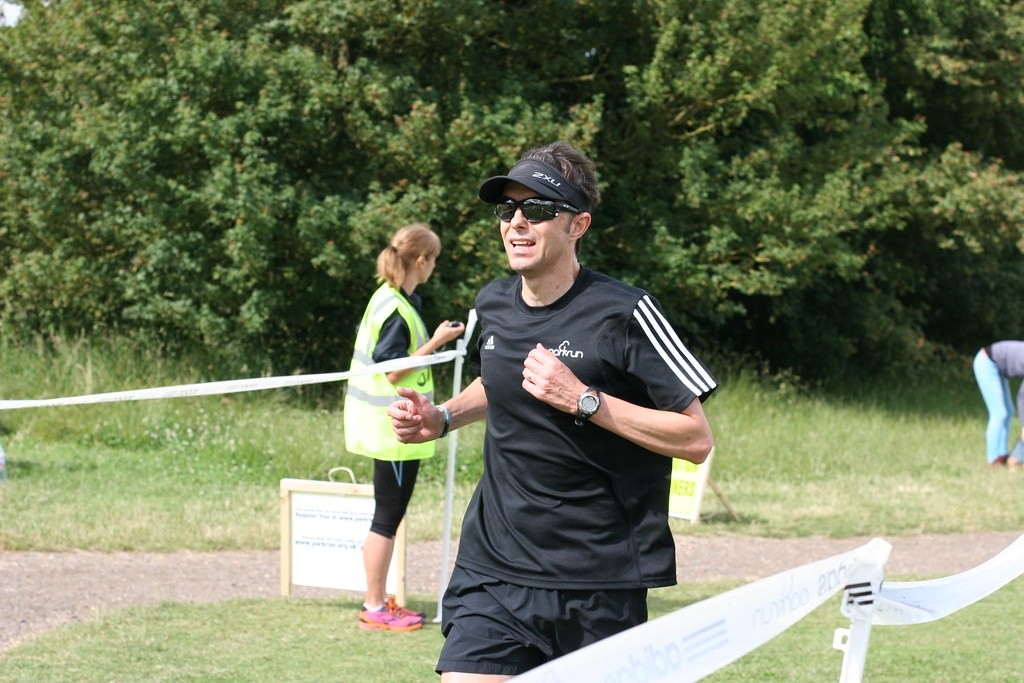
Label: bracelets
xmin=434 ymin=404 xmax=450 ymax=438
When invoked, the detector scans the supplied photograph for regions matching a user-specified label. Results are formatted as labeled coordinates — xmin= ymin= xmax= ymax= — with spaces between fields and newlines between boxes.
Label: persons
xmin=382 ymin=141 xmax=718 ymax=683
xmin=341 ymin=223 xmax=465 ymax=634
xmin=973 ymin=339 xmax=1024 ymax=467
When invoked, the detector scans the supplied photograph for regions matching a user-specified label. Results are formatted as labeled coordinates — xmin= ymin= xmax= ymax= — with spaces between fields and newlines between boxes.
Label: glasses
xmin=496 ymin=195 xmax=581 ymax=222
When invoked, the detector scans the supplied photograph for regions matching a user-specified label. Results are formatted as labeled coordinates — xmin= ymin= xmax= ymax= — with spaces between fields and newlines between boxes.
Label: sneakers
xmin=385 ymin=594 xmax=426 ymax=619
xmin=360 ymin=604 xmax=422 ymax=631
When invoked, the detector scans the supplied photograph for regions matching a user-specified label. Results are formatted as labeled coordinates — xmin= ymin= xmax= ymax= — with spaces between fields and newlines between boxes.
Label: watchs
xmin=573 ymin=385 xmax=603 ymax=426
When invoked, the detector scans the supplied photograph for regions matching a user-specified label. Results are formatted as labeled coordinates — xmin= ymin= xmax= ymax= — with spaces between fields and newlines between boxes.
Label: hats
xmin=479 ymin=158 xmax=592 ymax=215
